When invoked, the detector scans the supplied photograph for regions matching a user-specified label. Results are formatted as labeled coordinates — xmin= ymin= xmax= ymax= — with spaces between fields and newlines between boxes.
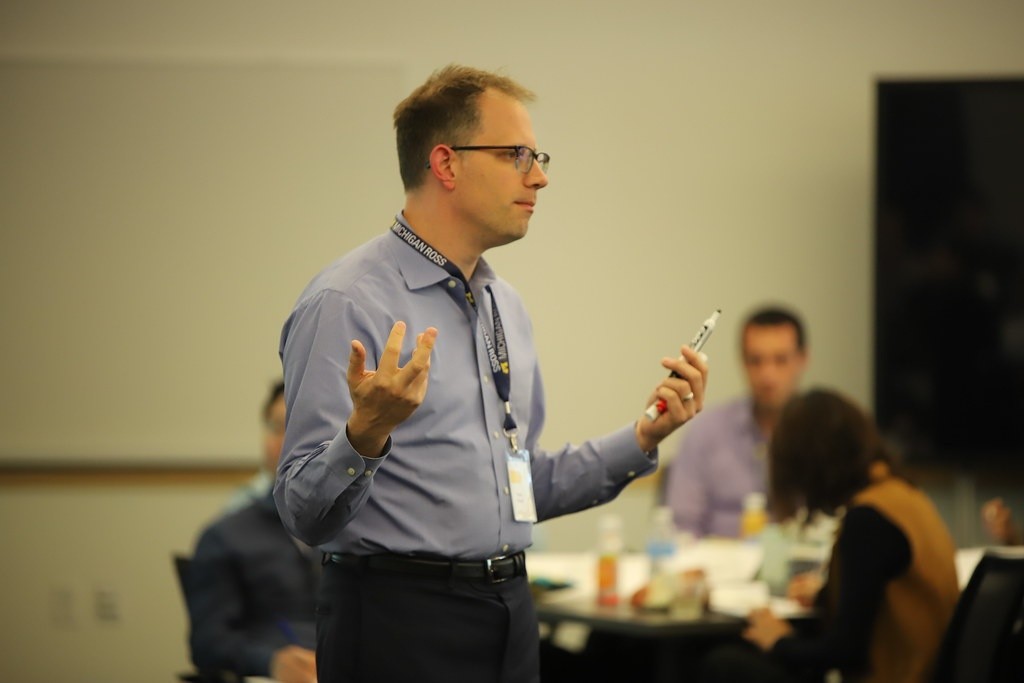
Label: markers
xmin=656 ymin=308 xmax=723 ymax=415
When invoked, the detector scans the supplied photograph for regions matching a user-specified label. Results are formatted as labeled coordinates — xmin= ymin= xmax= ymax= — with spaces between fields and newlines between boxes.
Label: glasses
xmin=425 ymin=143 xmax=552 ymax=176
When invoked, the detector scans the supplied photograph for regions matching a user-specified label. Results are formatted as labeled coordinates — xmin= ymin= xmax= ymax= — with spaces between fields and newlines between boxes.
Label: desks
xmin=532 ymin=575 xmax=962 ymax=683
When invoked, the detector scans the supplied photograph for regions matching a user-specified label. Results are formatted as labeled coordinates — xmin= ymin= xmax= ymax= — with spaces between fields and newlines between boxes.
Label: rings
xmin=682 ymin=392 xmax=694 ymax=402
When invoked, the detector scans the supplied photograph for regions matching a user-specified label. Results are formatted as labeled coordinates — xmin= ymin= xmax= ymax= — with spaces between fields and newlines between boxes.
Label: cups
xmin=666 ymin=569 xmax=704 ymax=624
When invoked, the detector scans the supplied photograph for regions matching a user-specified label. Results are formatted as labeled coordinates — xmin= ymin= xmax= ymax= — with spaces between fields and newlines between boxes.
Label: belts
xmin=322 ymin=551 xmax=526 ymax=584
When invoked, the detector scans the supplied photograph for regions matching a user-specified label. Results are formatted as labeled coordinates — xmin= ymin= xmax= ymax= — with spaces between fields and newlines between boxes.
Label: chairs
xmin=920 ymin=549 xmax=1023 ymax=681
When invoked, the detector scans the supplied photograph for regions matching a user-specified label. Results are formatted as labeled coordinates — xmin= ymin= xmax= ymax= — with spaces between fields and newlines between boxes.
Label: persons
xmin=742 ymin=384 xmax=962 ymax=683
xmin=184 ymin=381 xmax=317 ymax=683
xmin=273 ymin=61 xmax=711 ymax=683
xmin=669 ymin=305 xmax=810 ymax=537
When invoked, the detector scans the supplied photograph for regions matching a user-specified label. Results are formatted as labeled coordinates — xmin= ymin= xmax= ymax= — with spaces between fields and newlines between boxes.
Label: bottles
xmin=647 ymin=506 xmax=680 ymax=609
xmin=591 ymin=514 xmax=625 ymax=609
xmin=739 ymin=489 xmax=771 ymax=576
